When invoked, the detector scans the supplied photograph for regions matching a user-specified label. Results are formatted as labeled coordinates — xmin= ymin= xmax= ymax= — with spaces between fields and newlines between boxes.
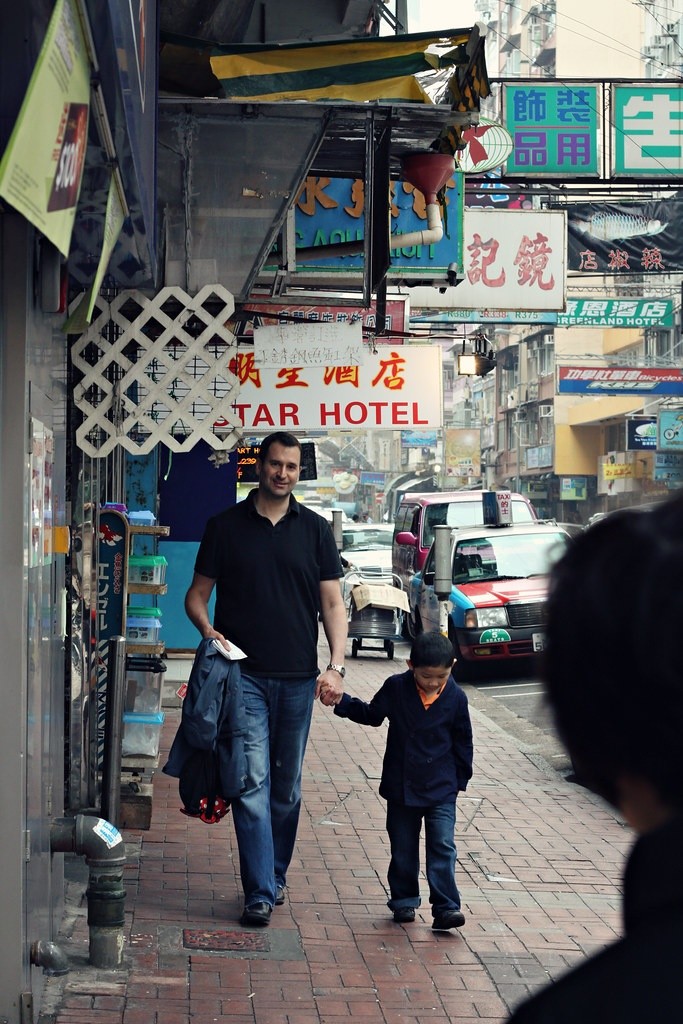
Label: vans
xmin=390 ymin=491 xmax=548 ymax=642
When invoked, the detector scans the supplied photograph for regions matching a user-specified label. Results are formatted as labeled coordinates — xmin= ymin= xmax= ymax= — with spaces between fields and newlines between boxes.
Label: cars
xmin=410 ymin=522 xmax=574 ymax=673
xmin=305 ymin=501 xmax=396 ymax=609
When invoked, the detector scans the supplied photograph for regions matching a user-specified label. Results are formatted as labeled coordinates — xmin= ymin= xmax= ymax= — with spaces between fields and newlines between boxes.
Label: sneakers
xmin=394 ymin=907 xmax=415 ymax=922
xmin=432 ymin=910 xmax=465 ymax=929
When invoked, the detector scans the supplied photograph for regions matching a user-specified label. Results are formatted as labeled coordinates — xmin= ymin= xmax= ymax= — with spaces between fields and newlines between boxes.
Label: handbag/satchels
xmin=179 ymin=754 xmax=234 ymax=824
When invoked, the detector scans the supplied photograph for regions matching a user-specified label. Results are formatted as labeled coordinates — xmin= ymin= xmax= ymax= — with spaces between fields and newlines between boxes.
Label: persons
xmin=184 ymin=432 xmax=348 ymax=925
xmin=503 ymin=485 xmax=682 ymax=1024
xmin=348 ymin=513 xmax=374 ymax=524
xmin=319 ymin=631 xmax=473 ymax=930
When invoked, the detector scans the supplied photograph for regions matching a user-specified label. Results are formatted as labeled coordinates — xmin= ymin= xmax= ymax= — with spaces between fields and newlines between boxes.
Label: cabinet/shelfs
xmin=120 ymin=525 xmax=170 ymax=778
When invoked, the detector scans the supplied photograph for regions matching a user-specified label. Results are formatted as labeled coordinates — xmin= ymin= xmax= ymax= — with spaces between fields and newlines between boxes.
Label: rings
xmin=332 ymin=701 xmax=336 ymax=704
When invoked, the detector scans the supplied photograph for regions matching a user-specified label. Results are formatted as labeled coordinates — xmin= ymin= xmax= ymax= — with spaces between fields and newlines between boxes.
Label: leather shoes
xmin=241 ymin=903 xmax=271 ymax=925
xmin=275 ymin=887 xmax=284 ymax=904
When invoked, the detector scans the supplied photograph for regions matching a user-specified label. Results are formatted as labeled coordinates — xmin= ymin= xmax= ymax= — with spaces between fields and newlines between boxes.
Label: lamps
xmin=457 ymin=340 xmax=498 ymax=378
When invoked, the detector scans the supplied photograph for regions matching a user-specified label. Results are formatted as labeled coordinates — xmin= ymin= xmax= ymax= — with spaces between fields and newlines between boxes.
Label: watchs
xmin=326 ymin=664 xmax=346 ymax=678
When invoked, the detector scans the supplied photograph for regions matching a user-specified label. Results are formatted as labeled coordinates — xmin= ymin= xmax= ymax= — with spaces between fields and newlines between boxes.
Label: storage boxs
xmin=127 ymin=606 xmax=162 ymax=618
xmin=126 ymin=617 xmax=163 ymax=646
xmin=125 ymin=657 xmax=168 ymax=714
xmin=123 ymin=712 xmax=165 ymax=757
xmin=129 ymin=555 xmax=169 ymax=585
